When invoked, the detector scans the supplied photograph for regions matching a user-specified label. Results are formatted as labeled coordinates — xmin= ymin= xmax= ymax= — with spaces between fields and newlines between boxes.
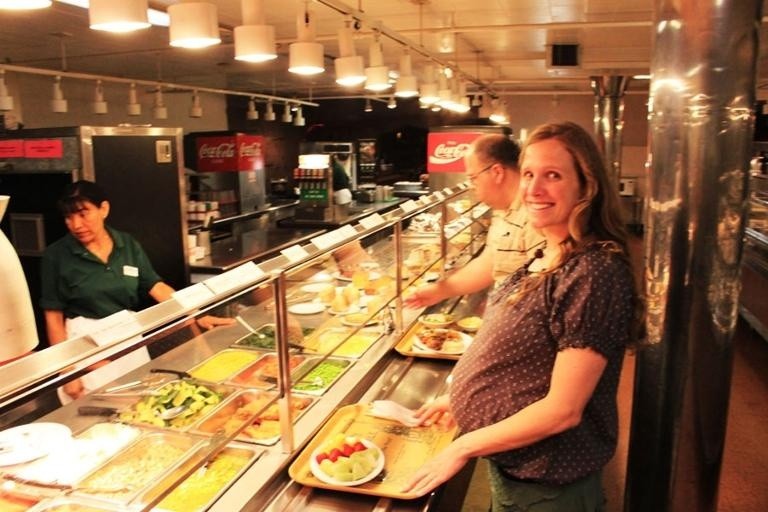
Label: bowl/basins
xmin=418 ymin=313 xmax=483 ymax=332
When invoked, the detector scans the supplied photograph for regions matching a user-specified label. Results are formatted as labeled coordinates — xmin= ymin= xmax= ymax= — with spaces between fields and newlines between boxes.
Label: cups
xmin=185 ymin=200 xmax=220 ymax=222
xmin=334 ymin=188 xmax=352 ymax=206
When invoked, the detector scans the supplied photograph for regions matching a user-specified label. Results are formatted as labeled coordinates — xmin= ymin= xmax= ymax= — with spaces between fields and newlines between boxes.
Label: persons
xmin=402 ymin=134 xmax=547 ymax=311
xmin=332 ymin=156 xmax=353 ymax=205
xmin=38 ymin=179 xmax=239 ymax=407
xmin=400 ymin=120 xmax=645 ymax=511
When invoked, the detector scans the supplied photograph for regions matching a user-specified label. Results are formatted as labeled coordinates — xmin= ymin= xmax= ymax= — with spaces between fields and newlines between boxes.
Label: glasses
xmin=465 ymin=162 xmax=506 ymax=185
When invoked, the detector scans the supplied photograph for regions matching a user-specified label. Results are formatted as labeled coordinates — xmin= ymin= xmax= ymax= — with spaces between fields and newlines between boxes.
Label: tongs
xmin=90 ymin=371 xmax=168 ymax=398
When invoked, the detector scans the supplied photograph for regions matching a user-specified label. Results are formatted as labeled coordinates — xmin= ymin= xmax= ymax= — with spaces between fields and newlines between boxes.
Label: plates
xmin=288 ymin=269 xmax=439 ymax=327
xmin=414 ymin=327 xmax=474 ymax=355
xmin=309 ymin=436 xmax=385 ymax=485
xmin=0 ymin=422 xmax=74 ymax=467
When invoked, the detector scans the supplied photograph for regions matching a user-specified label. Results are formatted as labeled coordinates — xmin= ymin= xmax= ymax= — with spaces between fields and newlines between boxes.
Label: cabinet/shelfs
xmin=748 ymin=142 xmax=768 ymax=237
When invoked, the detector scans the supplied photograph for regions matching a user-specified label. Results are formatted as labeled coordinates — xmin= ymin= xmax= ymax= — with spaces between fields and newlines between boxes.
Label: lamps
xmin=364 ymin=41 xmax=392 ymax=93
xmin=439 ymin=67 xmax=510 ymax=125
xmin=333 ymin=30 xmax=367 ymax=89
xmin=88 ymin=3 xmax=151 ymax=34
xmin=151 ymin=86 xmax=167 ymax=121
xmin=167 ymin=2 xmax=220 ymax=51
xmin=395 ymin=51 xmax=420 ymax=99
xmin=364 ymin=100 xmax=372 ymax=112
xmin=388 ymin=97 xmax=397 ymax=109
xmin=233 ymin=2 xmax=278 ymax=63
xmin=292 ymin=100 xmax=299 ymax=111
xmin=265 ymin=104 xmax=275 ymax=121
xmin=418 ymin=105 xmax=442 ymax=112
xmin=287 ymin=11 xmax=326 ymax=76
xmin=90 ymin=81 xmax=108 ymax=117
xmin=282 ymin=104 xmax=292 ymax=123
xmin=1 ymin=70 xmax=13 ymax=111
xmin=294 ymin=108 xmax=305 ymax=126
xmin=420 ymin=65 xmax=439 ymax=105
xmin=188 ymin=92 xmax=203 ymax=118
xmin=125 ymin=83 xmax=142 ymax=116
xmin=247 ymin=98 xmax=259 ymax=120
xmin=48 ymin=78 xmax=69 ymax=114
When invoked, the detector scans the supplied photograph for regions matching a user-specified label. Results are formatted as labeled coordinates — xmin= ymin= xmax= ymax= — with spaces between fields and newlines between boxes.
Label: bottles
xmin=374 ymin=185 xmax=391 ymax=202
